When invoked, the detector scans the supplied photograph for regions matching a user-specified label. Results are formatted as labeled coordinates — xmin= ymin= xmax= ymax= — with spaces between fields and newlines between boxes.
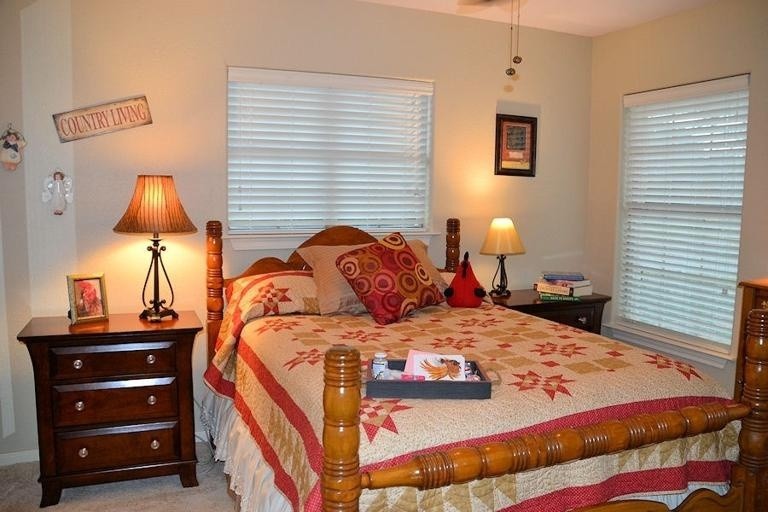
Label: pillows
xmin=225 ymin=230 xmax=451 ymax=326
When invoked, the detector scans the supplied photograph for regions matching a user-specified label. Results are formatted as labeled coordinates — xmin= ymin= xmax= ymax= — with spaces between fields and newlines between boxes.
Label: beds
xmin=200 ymin=220 xmax=768 ymax=511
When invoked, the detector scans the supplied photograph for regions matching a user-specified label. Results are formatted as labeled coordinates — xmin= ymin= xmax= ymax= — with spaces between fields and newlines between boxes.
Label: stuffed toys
xmin=443 ymin=252 xmax=486 ymax=307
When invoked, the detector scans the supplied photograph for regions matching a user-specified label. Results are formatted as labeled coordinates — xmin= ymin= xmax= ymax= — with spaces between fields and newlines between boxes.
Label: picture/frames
xmin=65 ymin=274 xmax=110 ymax=327
xmin=494 ymin=113 xmax=538 ymax=178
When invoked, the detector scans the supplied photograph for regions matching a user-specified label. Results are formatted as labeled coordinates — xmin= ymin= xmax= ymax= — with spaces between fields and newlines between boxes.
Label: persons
xmin=0 ymin=123 xmax=27 ymax=172
xmin=41 ymin=167 xmax=74 ymax=216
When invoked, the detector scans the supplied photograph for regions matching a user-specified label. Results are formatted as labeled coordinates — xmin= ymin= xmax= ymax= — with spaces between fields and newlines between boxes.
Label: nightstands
xmin=15 ymin=309 xmax=204 ymax=509
xmin=492 ymin=288 xmax=612 ymax=335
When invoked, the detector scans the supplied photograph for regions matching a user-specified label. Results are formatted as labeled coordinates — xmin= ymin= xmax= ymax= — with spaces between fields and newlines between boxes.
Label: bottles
xmin=373 ymin=352 xmax=389 ymax=377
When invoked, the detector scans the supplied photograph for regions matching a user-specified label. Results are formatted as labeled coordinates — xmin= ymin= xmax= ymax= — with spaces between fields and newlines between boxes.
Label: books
xmin=539 ymin=292 xmax=577 ymax=301
xmin=533 ymin=283 xmax=593 ymax=297
xmin=548 ymin=278 xmax=592 ymax=288
xmin=541 ymin=271 xmax=584 ymax=281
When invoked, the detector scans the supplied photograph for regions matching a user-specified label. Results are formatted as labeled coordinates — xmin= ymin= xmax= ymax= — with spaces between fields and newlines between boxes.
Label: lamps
xmin=113 ymin=174 xmax=198 ymax=322
xmin=478 ymin=217 xmax=527 ymax=299
